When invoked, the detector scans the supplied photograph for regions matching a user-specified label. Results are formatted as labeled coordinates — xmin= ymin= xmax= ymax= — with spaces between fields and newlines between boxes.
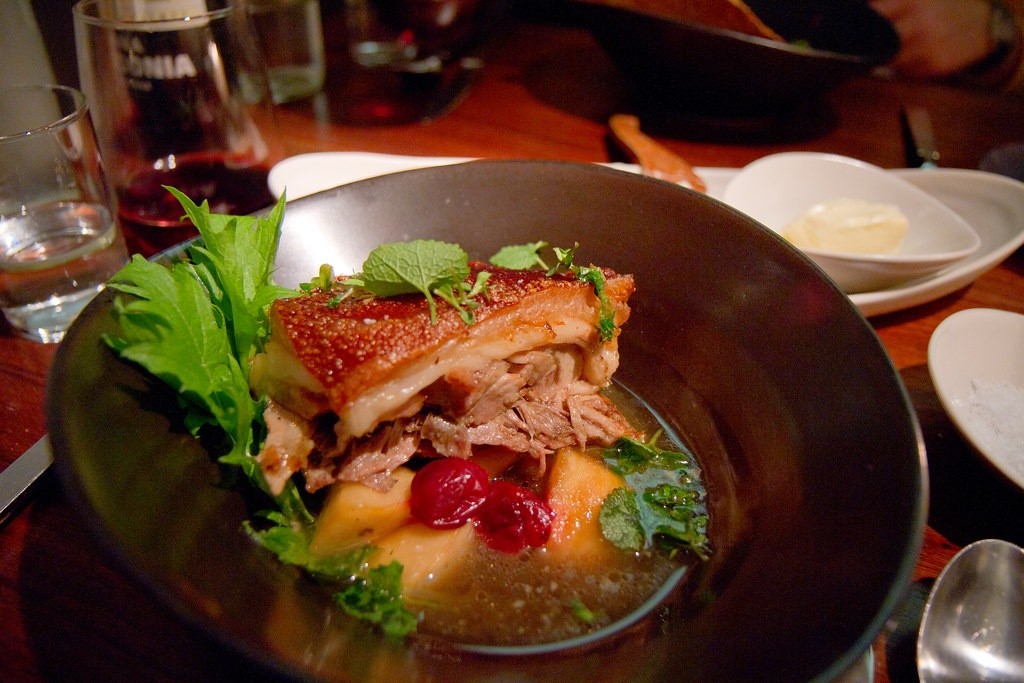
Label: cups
xmin=228 ymin=0 xmax=489 ymax=125
xmin=0 ymin=82 xmax=130 ymax=344
xmin=70 ymin=0 xmax=275 ymax=258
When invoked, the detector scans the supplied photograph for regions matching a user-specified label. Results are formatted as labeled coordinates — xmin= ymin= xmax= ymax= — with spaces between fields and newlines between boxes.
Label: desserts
xmin=771 ymin=199 xmax=907 ymax=259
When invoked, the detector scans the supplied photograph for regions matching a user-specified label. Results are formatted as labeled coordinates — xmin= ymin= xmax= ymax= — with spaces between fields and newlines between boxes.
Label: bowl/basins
xmin=581 ymin=0 xmax=902 ymax=109
xmin=721 ymin=151 xmax=980 ymax=293
xmin=44 ymin=157 xmax=930 ymax=683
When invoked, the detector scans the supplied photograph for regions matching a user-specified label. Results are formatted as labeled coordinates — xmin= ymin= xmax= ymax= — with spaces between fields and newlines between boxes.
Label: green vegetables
xmin=100 ymin=185 xmax=714 ymax=646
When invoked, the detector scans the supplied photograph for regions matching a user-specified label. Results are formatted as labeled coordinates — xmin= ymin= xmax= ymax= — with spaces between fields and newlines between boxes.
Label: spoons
xmin=916 ymin=538 xmax=1024 ymax=683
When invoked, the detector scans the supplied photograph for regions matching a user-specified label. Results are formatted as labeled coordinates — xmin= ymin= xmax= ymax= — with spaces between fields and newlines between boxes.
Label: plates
xmin=925 ymin=308 xmax=1024 ymax=490
xmin=267 ymin=145 xmax=1024 ymax=319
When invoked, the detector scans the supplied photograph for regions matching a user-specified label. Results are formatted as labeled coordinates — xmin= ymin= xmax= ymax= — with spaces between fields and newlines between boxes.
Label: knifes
xmin=906 ymin=105 xmax=940 ymax=168
xmin=0 ymin=435 xmax=52 ymax=523
